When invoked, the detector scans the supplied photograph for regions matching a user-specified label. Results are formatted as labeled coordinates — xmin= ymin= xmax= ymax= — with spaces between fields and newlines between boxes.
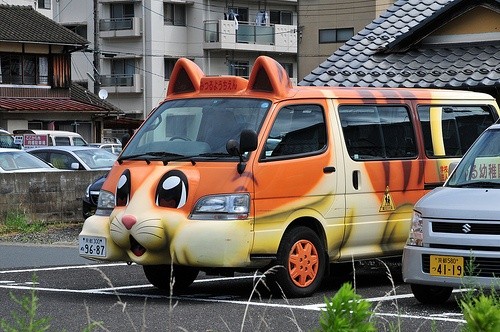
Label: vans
xmin=401 ymin=123 xmax=500 ymax=305
xmin=13 ymin=129 xmax=89 ymax=151
xmin=78 ymin=55 xmax=500 ymax=299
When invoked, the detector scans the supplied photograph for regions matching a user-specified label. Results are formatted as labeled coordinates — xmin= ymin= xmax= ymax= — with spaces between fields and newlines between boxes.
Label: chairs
xmin=204 ymin=111 xmax=235 ymax=150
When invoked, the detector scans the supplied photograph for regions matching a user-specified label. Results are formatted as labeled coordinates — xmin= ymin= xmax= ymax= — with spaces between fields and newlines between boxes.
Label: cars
xmin=0 ymin=129 xmax=26 ymax=150
xmin=2 ymin=146 xmax=118 ymax=171
xmin=0 ymin=147 xmax=58 ymax=171
xmin=88 ymin=142 xmax=123 ymax=157
